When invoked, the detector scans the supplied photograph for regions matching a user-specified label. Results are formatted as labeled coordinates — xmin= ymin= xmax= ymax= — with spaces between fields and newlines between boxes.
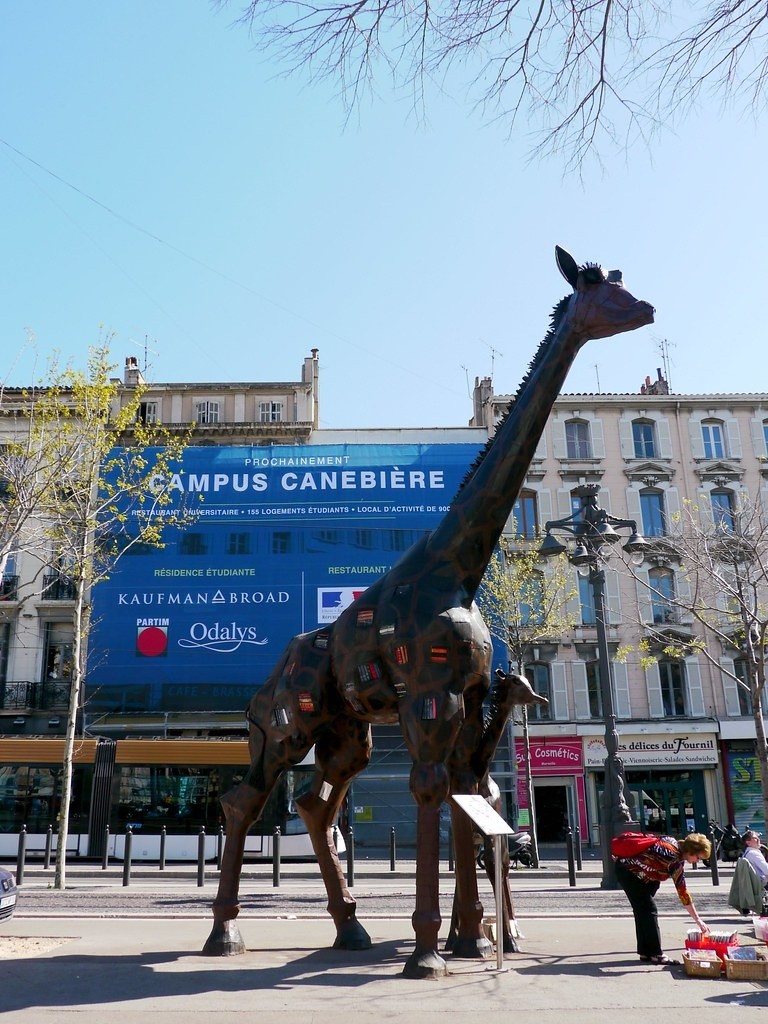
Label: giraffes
xmin=196 ymin=245 xmax=657 ymax=979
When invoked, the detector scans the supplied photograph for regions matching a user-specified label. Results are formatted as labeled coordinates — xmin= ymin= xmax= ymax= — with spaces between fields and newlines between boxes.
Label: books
xmin=687 ymin=927 xmax=756 ymax=962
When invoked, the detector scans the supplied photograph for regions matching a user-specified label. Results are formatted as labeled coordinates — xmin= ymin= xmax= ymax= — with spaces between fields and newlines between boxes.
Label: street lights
xmin=536 ymin=483 xmax=651 ymax=891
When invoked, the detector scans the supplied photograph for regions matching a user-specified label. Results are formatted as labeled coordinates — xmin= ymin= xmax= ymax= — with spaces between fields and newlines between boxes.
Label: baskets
xmin=724 ymin=952 xmax=768 ymax=981
xmin=682 ymin=949 xmax=723 ymax=977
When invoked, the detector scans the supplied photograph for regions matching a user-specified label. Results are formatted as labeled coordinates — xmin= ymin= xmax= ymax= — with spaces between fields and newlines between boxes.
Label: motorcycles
xmin=702 ymin=817 xmax=768 ymax=869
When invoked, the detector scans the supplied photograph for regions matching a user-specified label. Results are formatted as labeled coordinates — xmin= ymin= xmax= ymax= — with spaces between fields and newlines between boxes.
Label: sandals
xmin=650 ymin=953 xmax=681 ymax=966
xmin=640 ymin=954 xmax=651 ymax=963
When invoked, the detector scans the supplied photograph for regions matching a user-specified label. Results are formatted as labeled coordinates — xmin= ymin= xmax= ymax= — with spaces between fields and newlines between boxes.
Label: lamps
xmin=48 ymin=716 xmax=60 ymax=728
xmin=13 ymin=718 xmax=27 ymax=728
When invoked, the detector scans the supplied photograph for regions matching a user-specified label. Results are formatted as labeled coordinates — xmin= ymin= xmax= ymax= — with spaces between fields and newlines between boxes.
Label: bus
xmin=0 ymin=738 xmax=347 ymax=863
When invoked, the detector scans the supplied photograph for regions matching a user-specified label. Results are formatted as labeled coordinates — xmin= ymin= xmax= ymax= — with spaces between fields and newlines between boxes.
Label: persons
xmin=743 ymin=831 xmax=768 ymax=895
xmin=615 ymin=834 xmax=711 ymax=965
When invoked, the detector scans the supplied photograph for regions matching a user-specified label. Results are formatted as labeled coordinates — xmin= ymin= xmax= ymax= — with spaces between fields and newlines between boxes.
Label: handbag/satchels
xmin=611 ymin=831 xmax=666 ymax=858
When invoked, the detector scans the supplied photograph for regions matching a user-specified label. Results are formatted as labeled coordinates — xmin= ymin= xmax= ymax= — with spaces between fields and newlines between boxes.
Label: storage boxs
xmin=685 ymin=938 xmax=739 ymax=971
xmin=723 ymin=953 xmax=768 ymax=981
xmin=682 ymin=952 xmax=723 ymax=977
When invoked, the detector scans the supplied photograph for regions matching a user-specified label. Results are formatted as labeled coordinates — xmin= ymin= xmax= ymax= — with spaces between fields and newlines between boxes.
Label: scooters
xmin=477 ymin=832 xmax=532 ymax=871
